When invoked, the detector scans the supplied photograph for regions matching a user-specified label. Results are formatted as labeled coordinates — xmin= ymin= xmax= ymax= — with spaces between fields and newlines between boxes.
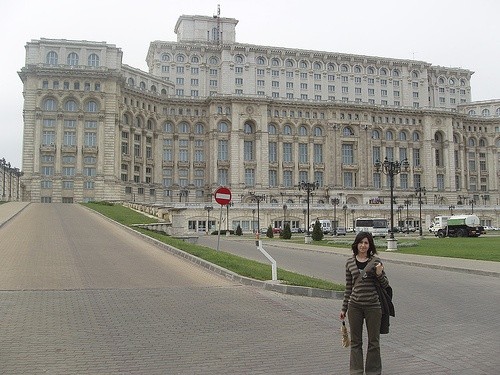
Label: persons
xmin=340 ymin=232 xmax=389 ymax=375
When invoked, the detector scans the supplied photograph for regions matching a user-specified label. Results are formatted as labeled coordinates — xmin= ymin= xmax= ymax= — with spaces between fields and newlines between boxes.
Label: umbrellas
xmin=341 ymin=321 xmax=349 ymax=348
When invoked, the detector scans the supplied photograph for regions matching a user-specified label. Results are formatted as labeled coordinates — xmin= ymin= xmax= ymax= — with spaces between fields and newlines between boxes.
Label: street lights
xmin=250 ymin=191 xmax=266 ymax=240
xmin=0 ymin=157 xmax=24 ymax=202
xmin=331 ymin=198 xmax=339 ymax=236
xmin=373 ymin=156 xmax=411 ymax=252
xmin=298 ymin=180 xmax=320 ymax=244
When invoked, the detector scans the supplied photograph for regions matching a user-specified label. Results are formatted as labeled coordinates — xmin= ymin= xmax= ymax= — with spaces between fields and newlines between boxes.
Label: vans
xmin=308 ymin=219 xmax=331 ymax=235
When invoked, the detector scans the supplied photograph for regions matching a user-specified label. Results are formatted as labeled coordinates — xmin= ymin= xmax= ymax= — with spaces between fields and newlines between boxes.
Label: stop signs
xmin=214 ymin=186 xmax=232 ymax=206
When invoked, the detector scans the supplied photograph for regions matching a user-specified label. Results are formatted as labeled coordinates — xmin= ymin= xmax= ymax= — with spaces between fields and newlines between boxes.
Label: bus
xmin=355 ymin=217 xmax=388 ymax=238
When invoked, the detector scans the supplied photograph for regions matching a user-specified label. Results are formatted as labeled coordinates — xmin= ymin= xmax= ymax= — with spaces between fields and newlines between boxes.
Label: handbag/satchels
xmin=387 ymin=285 xmax=392 ymax=300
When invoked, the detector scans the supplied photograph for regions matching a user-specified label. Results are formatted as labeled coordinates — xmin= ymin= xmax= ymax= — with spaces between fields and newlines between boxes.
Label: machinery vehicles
xmin=433 ymin=215 xmax=485 ymax=237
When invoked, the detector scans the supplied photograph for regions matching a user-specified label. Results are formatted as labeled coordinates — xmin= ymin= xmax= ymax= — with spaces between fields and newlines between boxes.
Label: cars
xmin=388 ymin=224 xmax=416 ymax=232
xmin=261 ymin=227 xmax=305 ymax=233
xmin=330 ymin=227 xmax=346 ymax=236
xmin=483 ymin=225 xmax=500 ymax=231
xmin=346 ymin=227 xmax=354 ymax=232
